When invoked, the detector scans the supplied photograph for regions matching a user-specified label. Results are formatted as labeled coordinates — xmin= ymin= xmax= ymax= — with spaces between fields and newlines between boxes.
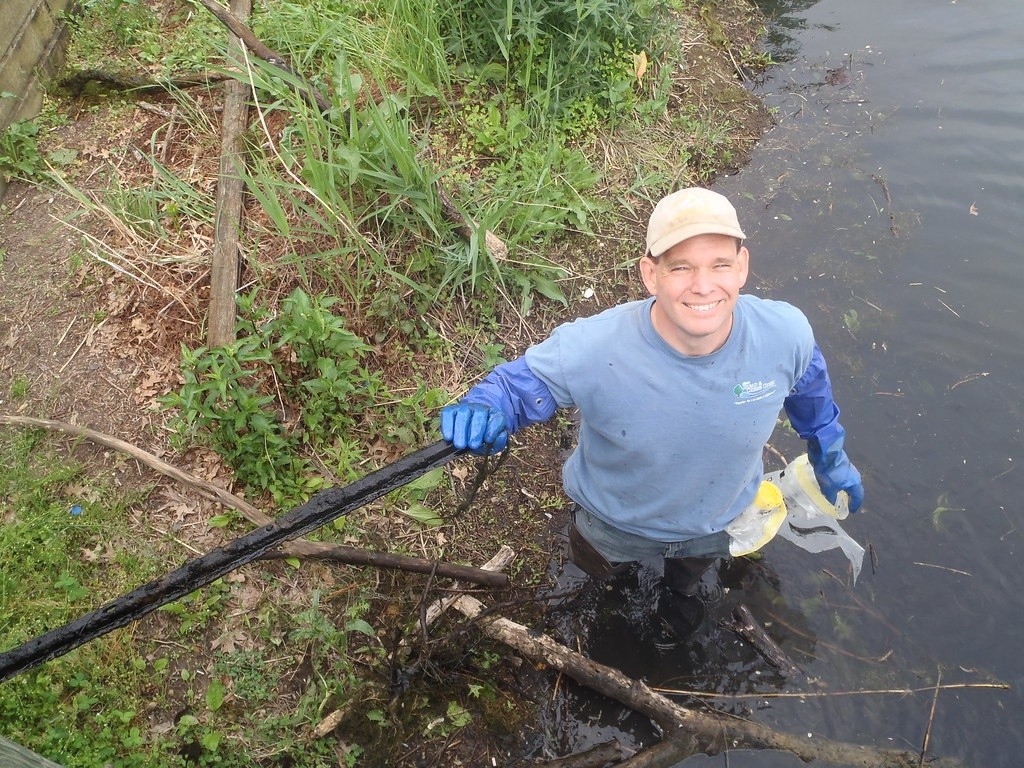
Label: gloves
xmin=442 ymin=354 xmax=558 ymax=456
xmin=783 ymin=342 xmax=865 ymax=513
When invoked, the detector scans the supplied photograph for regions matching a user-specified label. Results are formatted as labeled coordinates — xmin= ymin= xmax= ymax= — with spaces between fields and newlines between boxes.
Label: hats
xmin=646 ymin=187 xmax=747 ymax=258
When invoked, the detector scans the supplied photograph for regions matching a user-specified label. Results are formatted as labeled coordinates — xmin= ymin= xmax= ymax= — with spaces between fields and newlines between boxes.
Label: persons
xmin=439 ymin=187 xmax=865 ymax=596
